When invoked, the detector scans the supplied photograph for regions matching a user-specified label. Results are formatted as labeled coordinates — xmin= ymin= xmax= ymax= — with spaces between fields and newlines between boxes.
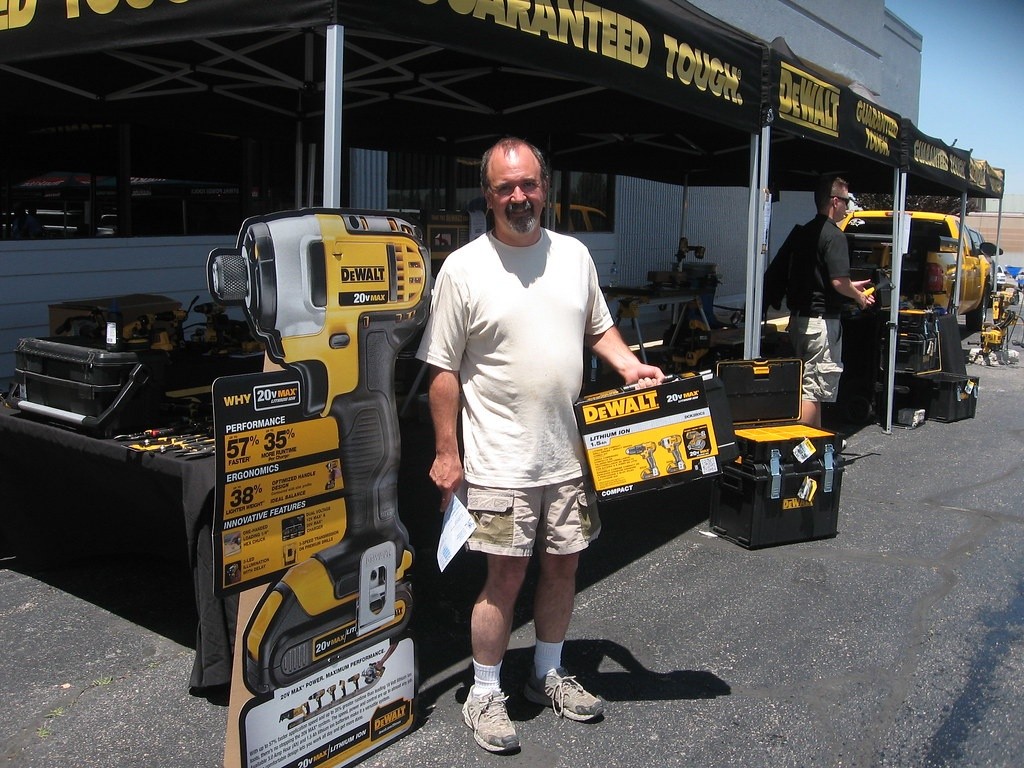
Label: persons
xmin=414 ymin=137 xmax=665 ymax=751
xmin=784 ymin=175 xmax=875 ymax=451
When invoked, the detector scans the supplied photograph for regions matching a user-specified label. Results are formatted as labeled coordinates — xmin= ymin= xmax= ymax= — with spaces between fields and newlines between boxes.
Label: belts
xmin=791 ymin=309 xmax=840 ymax=319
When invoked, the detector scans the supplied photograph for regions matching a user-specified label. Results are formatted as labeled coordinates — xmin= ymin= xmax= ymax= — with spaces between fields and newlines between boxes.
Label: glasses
xmin=827 ymin=194 xmax=850 ymax=206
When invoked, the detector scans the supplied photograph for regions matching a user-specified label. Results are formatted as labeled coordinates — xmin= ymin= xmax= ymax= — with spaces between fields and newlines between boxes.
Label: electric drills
xmin=194 ymin=302 xmax=224 ymax=343
xmin=154 ymin=309 xmax=188 ymax=344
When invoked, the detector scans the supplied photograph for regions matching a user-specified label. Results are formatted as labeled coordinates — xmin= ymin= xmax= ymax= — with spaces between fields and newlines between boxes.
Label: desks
xmin=605 ymin=290 xmax=711 ymax=372
xmin=1 ymin=410 xmax=242 ymax=694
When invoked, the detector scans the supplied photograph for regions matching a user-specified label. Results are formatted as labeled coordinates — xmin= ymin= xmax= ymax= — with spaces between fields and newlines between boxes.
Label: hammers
xmin=862 ymin=272 xmax=896 ymax=299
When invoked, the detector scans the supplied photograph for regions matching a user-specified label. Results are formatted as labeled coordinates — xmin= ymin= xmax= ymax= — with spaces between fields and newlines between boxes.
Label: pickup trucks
xmin=840 ymin=209 xmax=1004 ymax=332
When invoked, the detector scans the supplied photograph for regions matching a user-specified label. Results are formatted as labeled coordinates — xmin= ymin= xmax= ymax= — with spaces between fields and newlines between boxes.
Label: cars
xmin=997 ymin=265 xmax=1007 ymax=286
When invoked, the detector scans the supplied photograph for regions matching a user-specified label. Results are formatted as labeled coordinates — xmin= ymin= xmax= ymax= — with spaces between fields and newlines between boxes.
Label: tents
xmin=0 ymin=0 xmax=1005 ymax=434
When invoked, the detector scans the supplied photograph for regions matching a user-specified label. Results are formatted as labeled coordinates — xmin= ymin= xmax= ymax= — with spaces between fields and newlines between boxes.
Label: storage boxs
xmin=576 ymin=330 xmax=848 ymax=550
xmin=849 ymin=268 xmax=979 ymax=422
xmin=14 ymin=294 xmax=182 ymax=430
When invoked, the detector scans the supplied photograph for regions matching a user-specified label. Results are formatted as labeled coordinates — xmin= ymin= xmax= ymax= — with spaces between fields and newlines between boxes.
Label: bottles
xmin=106 ymin=312 xmax=123 ymax=352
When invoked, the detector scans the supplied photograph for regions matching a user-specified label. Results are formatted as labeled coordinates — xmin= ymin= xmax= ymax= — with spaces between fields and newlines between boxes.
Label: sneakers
xmin=524 ymin=664 xmax=604 ymax=720
xmin=463 ymin=685 xmax=521 ymax=751
